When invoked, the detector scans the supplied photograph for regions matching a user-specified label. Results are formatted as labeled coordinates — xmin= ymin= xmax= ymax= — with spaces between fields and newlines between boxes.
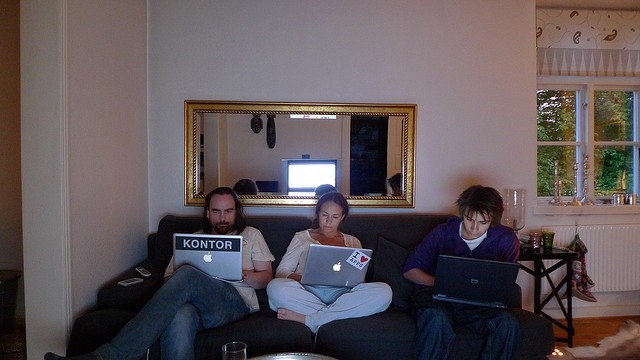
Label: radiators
xmin=540 ymin=224 xmax=640 ymax=318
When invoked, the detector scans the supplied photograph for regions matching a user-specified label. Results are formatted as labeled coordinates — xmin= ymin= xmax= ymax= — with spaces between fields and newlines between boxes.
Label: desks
xmin=519 ymin=247 xmax=577 ymax=348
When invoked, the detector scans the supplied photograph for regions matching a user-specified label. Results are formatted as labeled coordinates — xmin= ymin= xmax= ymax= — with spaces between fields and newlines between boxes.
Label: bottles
xmin=626 ymin=194 xmax=637 ymax=205
xmin=613 ymin=193 xmax=625 ymax=205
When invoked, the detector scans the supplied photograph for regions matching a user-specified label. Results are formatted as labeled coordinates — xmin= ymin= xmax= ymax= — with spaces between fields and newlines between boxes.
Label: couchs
xmin=70 ymin=213 xmax=554 ymax=359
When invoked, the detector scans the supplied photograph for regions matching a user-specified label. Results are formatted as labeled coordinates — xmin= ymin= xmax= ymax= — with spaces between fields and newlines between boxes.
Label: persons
xmin=402 ymin=184 xmax=524 ymax=359
xmin=44 ymin=187 xmax=276 ymax=359
xmin=264 ymin=193 xmax=392 ymax=332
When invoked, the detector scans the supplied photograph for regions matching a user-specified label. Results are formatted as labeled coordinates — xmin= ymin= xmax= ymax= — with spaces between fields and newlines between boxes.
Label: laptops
xmin=432 ymin=254 xmax=520 ymax=310
xmin=171 ymin=233 xmax=244 ymax=282
xmin=300 ymin=244 xmax=373 ymax=288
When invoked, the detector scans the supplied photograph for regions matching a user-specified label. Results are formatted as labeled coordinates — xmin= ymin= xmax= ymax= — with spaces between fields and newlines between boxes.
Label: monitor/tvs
xmin=287 ymin=161 xmax=338 ymax=192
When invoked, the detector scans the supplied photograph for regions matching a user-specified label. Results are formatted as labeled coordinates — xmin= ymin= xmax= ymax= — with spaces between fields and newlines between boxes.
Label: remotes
xmin=135 ymin=266 xmax=151 ymax=278
xmin=117 ymin=276 xmax=143 ymax=287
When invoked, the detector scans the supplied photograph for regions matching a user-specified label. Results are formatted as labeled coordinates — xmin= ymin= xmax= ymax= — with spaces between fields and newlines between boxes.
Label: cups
xmin=222 ymin=340 xmax=247 ymax=360
xmin=529 ymin=231 xmax=541 ymax=252
xmin=542 ymin=231 xmax=555 ymax=251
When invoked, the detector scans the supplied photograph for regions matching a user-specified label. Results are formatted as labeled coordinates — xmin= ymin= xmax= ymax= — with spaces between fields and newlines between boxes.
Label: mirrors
xmin=183 ymin=99 xmax=416 ymax=208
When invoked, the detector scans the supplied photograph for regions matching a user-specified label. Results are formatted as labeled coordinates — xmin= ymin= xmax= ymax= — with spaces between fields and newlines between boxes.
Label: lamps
xmin=503 ymin=188 xmax=526 ymax=244
